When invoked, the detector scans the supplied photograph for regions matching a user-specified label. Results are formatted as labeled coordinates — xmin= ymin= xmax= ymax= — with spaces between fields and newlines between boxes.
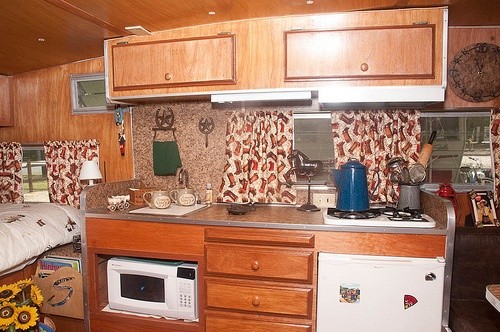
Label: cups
xmin=170 ymin=188 xmax=196 ymax=206
xmin=396 ymin=185 xmax=421 ymax=211
xmin=143 ymin=190 xmax=171 ymax=209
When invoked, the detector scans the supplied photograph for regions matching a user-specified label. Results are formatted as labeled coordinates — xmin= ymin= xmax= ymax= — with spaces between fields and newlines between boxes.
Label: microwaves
xmin=106 ymin=255 xmax=199 ymax=321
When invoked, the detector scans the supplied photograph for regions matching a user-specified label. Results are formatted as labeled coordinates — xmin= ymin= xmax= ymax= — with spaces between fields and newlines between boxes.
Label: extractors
xmin=317 ymin=87 xmax=445 ymax=109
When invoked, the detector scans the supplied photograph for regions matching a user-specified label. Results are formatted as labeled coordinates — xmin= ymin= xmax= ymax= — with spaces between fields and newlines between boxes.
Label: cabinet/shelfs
xmin=109 ymin=8 xmax=443 ymax=98
xmin=202 ymin=228 xmax=315 ymax=332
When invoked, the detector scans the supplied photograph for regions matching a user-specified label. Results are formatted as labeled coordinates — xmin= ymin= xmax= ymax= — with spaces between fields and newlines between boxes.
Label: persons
xmin=477 ymin=200 xmax=492 ymax=223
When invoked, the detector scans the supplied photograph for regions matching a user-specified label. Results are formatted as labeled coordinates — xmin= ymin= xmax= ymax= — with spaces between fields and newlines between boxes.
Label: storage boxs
xmin=31 ymin=266 xmax=84 ymax=319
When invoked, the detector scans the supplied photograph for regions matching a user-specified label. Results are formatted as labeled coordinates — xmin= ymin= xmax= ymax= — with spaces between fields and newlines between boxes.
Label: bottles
xmin=436 ymin=182 xmax=455 ymax=202
xmin=206 ymin=183 xmax=213 ymax=206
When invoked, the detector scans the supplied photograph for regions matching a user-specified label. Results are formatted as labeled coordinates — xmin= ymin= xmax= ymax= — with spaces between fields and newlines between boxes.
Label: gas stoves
xmin=324 ymin=207 xmax=437 ymax=228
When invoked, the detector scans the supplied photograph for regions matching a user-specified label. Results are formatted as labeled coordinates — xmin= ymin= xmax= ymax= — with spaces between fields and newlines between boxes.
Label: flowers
xmin=0 ymin=278 xmax=43 ymax=332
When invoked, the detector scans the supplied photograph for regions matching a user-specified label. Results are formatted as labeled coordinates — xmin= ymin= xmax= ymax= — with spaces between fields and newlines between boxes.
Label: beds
xmin=0 ymin=200 xmax=81 ymax=285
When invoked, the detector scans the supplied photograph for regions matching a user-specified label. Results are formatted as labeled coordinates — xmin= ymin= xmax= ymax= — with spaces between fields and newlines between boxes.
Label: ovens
xmin=313 ymin=252 xmax=447 ymax=332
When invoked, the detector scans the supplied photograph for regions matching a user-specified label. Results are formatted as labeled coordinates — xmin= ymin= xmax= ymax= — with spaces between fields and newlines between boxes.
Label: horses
xmin=283 ymin=149 xmax=336 ymax=188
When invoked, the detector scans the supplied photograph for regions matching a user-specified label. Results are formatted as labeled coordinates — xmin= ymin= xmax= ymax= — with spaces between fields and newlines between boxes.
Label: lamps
xmin=78 ymin=159 xmax=102 ymax=185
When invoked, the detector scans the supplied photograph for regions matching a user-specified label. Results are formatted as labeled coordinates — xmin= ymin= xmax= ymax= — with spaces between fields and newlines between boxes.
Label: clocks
xmin=450 ymin=43 xmax=500 ymax=102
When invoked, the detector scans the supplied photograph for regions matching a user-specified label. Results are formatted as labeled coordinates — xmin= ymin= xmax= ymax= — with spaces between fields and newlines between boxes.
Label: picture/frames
xmin=468 ymin=190 xmax=500 ymax=228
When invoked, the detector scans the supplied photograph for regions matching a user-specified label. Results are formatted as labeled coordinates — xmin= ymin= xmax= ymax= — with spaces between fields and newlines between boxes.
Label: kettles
xmin=330 ymin=158 xmax=370 ymax=212
